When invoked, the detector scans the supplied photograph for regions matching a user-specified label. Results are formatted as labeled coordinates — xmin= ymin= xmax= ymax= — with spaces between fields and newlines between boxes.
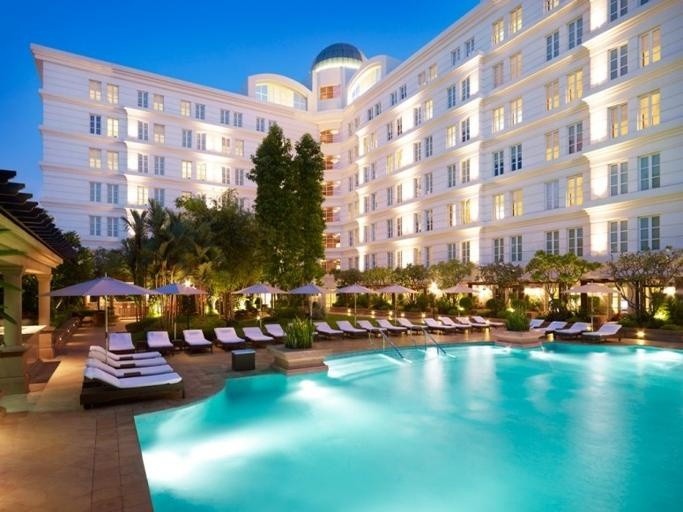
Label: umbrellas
xmin=560 ymin=285 xmax=620 ymax=332
xmin=231 ymin=284 xmax=289 ymax=329
xmin=375 ymin=286 xmax=423 ymax=325
xmin=335 ymin=285 xmax=376 ymax=329
xmin=440 ymin=285 xmax=481 ymax=317
xmin=153 ymin=284 xmax=210 ymax=341
xmin=287 ymin=285 xmax=335 ymax=327
xmin=36 ymin=277 xmax=165 ymax=352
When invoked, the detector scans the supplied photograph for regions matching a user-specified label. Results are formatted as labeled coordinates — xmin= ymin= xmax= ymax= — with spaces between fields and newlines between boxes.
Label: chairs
xmin=528 ymin=318 xmax=623 ymax=343
xmin=80 ymin=344 xmax=185 ymax=412
xmin=105 ymin=323 xmax=292 ymax=356
xmin=313 ymin=315 xmax=506 ymax=337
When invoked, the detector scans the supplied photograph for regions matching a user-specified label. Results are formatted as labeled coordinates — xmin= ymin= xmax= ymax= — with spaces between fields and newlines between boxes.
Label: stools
xmin=231 ymin=348 xmax=257 ymax=371
